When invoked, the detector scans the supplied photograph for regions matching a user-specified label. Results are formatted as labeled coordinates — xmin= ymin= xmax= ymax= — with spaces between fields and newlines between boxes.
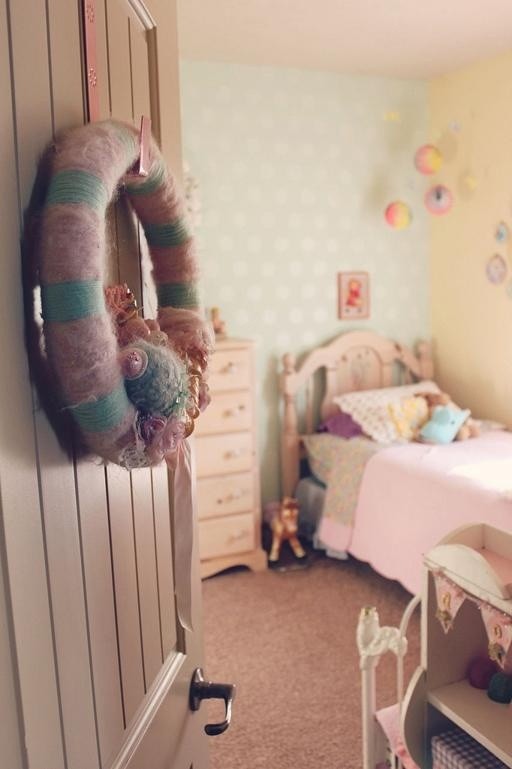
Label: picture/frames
xmin=338 ymin=270 xmax=371 ymax=319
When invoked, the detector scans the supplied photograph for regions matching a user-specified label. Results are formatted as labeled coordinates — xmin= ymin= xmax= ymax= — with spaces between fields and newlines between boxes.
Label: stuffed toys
xmin=389 ymin=389 xmax=483 ymax=444
xmin=263 ymin=494 xmax=311 ymax=572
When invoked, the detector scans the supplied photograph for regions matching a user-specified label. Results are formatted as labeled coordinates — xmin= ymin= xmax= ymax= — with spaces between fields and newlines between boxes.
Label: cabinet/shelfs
xmin=188 ymin=333 xmax=268 ymax=579
xmin=355 ymin=521 xmax=512 ymax=769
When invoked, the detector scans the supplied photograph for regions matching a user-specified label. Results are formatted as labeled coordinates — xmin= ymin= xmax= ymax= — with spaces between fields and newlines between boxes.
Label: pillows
xmin=318 ymin=410 xmax=363 ymax=439
xmin=334 ymin=381 xmax=463 ymax=444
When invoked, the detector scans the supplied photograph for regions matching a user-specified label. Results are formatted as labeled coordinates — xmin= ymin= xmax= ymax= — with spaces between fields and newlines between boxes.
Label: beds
xmin=280 ymin=329 xmax=512 ymax=607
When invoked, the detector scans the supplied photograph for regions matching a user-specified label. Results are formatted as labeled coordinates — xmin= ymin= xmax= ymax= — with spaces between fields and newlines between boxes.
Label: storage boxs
xmin=430 ymin=728 xmax=506 ymax=769
